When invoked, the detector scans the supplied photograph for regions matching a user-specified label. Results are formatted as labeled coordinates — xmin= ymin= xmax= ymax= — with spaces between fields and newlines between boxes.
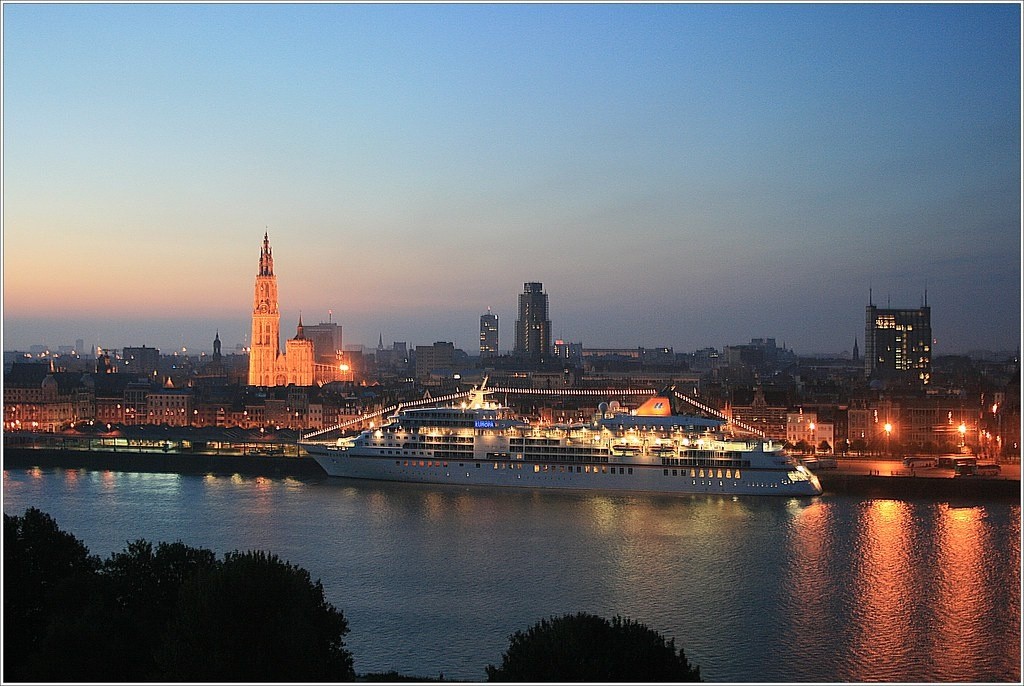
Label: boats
xmin=296 ymin=387 xmax=822 ymax=495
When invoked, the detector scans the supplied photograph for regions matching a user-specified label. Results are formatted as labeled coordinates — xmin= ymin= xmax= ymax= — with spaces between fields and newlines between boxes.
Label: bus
xmin=940 ymin=457 xmax=1002 ymax=476
xmin=903 ymin=457 xmax=936 ymax=468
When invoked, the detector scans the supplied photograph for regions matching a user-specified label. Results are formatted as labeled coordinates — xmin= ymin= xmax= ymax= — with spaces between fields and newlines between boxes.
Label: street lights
xmin=884 ymin=424 xmax=891 ymax=452
